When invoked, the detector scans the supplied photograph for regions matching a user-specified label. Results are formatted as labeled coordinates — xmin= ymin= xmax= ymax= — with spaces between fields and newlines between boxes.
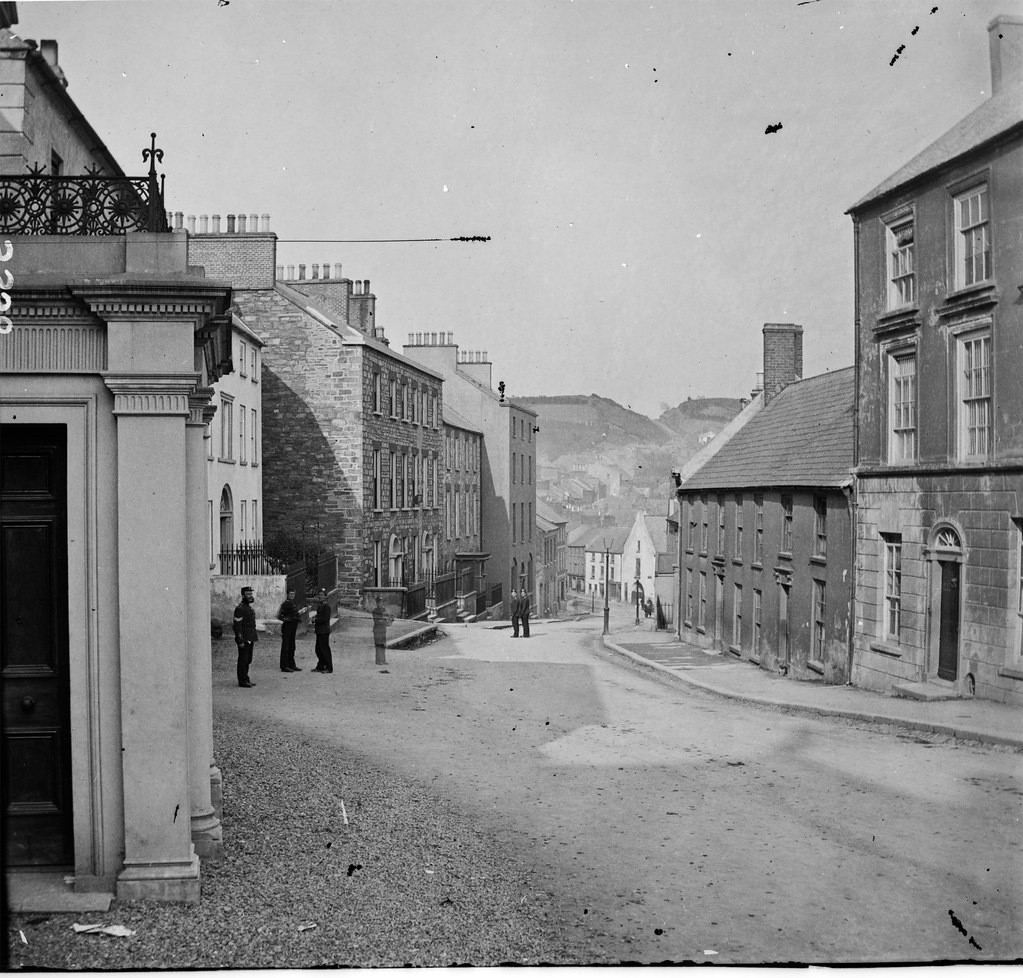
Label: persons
xmin=521 ymin=588 xmax=531 ymax=638
xmin=232 ymin=586 xmax=259 ymax=688
xmin=276 ymin=587 xmax=303 ymax=673
xmin=372 ymin=596 xmax=392 ymax=666
xmin=509 ymin=589 xmax=520 ymax=639
xmin=312 ymin=588 xmax=334 ymax=674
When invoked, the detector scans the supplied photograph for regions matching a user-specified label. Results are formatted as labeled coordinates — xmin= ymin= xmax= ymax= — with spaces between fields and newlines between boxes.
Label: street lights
xmin=634 ymin=576 xmax=641 ymax=626
xmin=601 ymin=538 xmax=615 ymax=636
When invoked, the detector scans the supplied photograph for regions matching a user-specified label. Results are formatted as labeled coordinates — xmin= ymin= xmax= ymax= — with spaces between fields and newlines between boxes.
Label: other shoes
xmin=519 ymin=635 xmax=529 ymax=638
xmin=289 ymin=666 xmax=302 ymax=672
xmin=281 ymin=667 xmax=294 ymax=673
xmin=511 ymin=635 xmax=518 ymax=638
xmin=311 ymin=668 xmax=323 ymax=673
xmin=322 ymin=669 xmax=333 ymax=674
xmin=238 ymin=682 xmax=257 ymax=687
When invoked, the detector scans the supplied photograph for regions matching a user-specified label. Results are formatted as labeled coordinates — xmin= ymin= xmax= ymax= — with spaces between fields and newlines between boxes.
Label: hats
xmin=375 ymin=597 xmax=383 ymax=603
xmin=241 ymin=586 xmax=254 ymax=592
xmin=511 ymin=589 xmax=517 ymax=593
xmin=318 ymin=588 xmax=328 ymax=597
xmin=521 ymin=588 xmax=525 ymax=592
xmin=287 ymin=588 xmax=295 ymax=594
